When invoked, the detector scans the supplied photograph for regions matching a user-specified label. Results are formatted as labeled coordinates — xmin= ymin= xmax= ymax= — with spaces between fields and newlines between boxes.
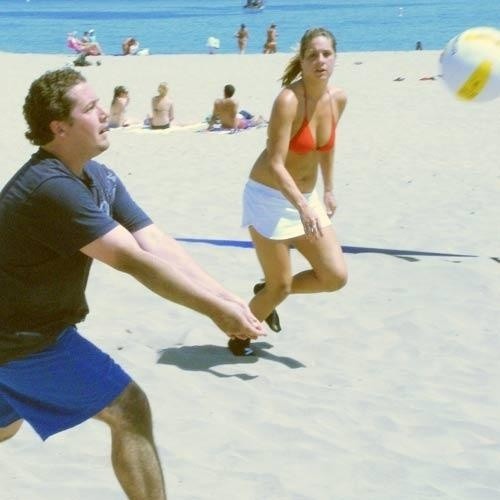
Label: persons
xmin=72 ymin=30 xmax=101 ymax=56
xmin=0 ymin=66 xmax=268 ymax=500
xmin=233 ymin=23 xmax=249 ymax=55
xmin=264 ymin=21 xmax=277 ymax=54
xmin=122 ymin=38 xmax=140 ymax=56
xmin=202 ymin=85 xmax=262 ymax=133
xmin=81 ymin=30 xmax=97 ymax=56
xmin=227 ymin=27 xmax=349 ymax=357
xmin=105 ymin=85 xmax=130 ymax=128
xmin=148 ymin=81 xmax=174 ymax=129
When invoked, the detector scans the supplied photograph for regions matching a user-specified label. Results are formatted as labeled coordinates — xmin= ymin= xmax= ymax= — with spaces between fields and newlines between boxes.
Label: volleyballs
xmin=440 ymin=25 xmax=500 ymax=103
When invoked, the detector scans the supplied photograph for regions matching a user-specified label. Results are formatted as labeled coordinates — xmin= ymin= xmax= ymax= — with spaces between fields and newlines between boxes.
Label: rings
xmin=312 ymin=224 xmax=316 ymax=229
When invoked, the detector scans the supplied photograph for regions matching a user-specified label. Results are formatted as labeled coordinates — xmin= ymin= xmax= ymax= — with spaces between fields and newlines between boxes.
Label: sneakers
xmin=226 ymin=335 xmax=257 ymax=358
xmin=253 ymin=281 xmax=283 ymax=334
